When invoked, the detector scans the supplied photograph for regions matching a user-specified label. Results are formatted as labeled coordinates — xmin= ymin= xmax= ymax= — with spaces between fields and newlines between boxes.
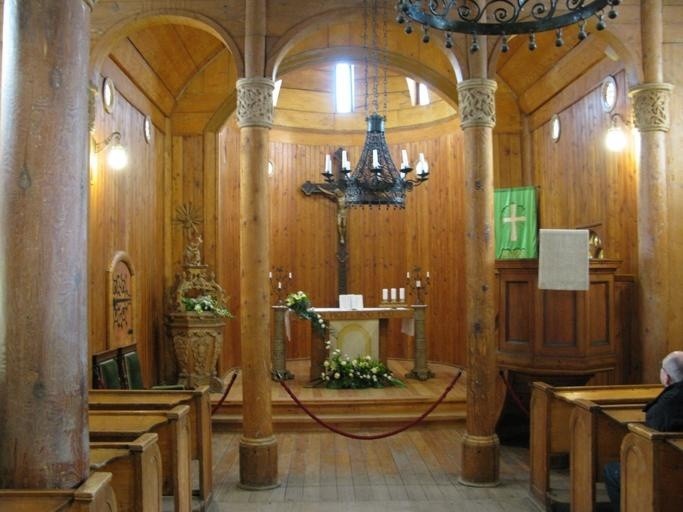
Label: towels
xmin=536 ymin=228 xmax=590 ymax=292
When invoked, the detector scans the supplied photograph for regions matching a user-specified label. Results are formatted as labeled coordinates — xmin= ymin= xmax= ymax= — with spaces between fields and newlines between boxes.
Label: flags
xmin=493 ymin=185 xmax=541 ymax=260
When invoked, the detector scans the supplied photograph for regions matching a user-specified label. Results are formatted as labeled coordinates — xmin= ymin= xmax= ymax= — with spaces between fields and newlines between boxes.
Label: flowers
xmin=285 ymin=290 xmax=312 ymax=320
xmin=314 ymin=314 xmax=407 ymax=389
xmin=180 ymin=295 xmax=239 ymax=320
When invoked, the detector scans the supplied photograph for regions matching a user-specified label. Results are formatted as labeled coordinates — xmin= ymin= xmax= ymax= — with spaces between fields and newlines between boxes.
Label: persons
xmin=606 ymin=350 xmax=683 ymax=511
xmin=316 ymin=184 xmax=350 ymax=244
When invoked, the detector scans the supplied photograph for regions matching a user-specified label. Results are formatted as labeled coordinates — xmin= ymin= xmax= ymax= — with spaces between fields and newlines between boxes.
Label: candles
xmin=269 ymin=272 xmax=292 ymax=288
xmin=382 ymin=289 xmax=388 ymax=300
xmin=391 ymin=288 xmax=397 ymax=300
xmin=407 ymin=272 xmax=429 ymax=287
xmin=399 ymin=287 xmax=406 ymax=300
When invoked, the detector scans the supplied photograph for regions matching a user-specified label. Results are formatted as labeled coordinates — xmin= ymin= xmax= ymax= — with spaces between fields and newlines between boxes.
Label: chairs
xmin=91 ymin=349 xmax=127 ymax=389
xmin=117 ymin=343 xmax=195 ymax=390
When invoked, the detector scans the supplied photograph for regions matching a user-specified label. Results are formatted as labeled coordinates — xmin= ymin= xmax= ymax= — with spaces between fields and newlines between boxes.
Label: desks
xmin=0 ymin=384 xmax=213 ymax=512
xmin=529 ymin=381 xmax=683 ymax=512
xmin=297 ymin=307 xmax=416 ymax=388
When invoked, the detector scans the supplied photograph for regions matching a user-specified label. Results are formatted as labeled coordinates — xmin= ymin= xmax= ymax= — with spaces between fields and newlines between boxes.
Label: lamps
xmin=606 ymin=114 xmax=633 ymax=152
xmin=395 ymin=0 xmax=621 ymax=54
xmin=320 ymin=0 xmax=430 ymax=211
xmin=89 ymin=131 xmax=128 ymax=185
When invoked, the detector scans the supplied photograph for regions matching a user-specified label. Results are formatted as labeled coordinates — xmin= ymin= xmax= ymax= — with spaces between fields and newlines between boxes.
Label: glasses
xmin=660 ymin=362 xmax=668 ymax=375
